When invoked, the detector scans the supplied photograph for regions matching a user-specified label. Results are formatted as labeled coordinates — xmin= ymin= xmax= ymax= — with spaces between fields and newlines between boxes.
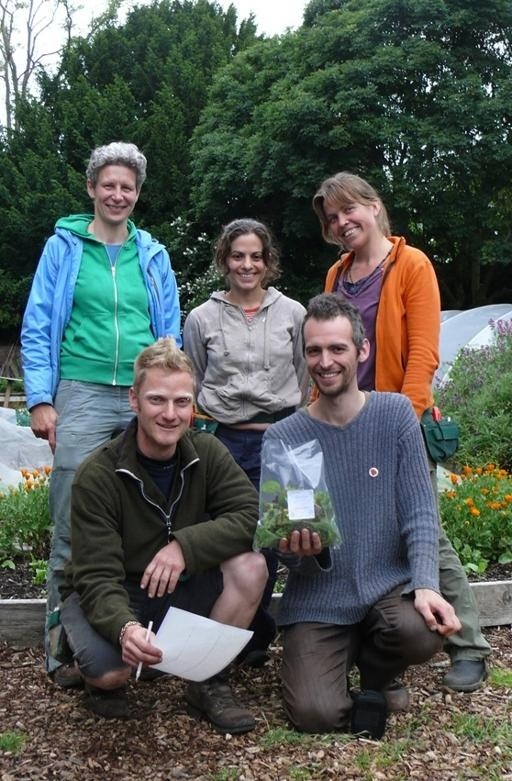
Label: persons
xmin=53 ymin=336 xmax=272 ymax=740
xmin=311 ymin=171 xmax=495 ymax=696
xmin=179 ymin=218 xmax=316 ymax=669
xmin=18 ymin=142 xmax=185 ymax=690
xmin=252 ymin=292 xmax=463 ymax=737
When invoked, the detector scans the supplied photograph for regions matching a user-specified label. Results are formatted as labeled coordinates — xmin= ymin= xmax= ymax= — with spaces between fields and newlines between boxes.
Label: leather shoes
xmin=443 ymin=660 xmax=488 ymax=691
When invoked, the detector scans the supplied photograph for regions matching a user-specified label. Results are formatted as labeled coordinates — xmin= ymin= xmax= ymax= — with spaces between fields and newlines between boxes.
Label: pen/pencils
xmin=135 ymin=620 xmax=154 ymax=682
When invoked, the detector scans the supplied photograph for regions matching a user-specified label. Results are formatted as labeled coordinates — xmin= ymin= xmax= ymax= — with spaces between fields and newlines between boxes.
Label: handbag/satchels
xmin=420 ymin=409 xmax=459 ymax=463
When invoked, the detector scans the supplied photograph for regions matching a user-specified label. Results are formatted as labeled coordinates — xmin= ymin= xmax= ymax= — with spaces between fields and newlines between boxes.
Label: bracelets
xmin=119 ymin=621 xmax=145 ymax=646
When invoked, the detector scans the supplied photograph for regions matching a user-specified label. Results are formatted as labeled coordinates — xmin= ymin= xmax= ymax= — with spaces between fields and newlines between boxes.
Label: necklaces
xmin=346 ymin=265 xmax=356 ymax=293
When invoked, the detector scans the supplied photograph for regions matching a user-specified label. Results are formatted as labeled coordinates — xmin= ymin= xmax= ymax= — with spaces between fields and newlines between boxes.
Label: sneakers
xmin=184 ymin=679 xmax=256 ymax=734
xmin=381 ymin=677 xmax=409 ymax=714
xmin=86 ymin=684 xmax=128 ymax=716
xmin=50 ymin=663 xmax=83 ymax=686
